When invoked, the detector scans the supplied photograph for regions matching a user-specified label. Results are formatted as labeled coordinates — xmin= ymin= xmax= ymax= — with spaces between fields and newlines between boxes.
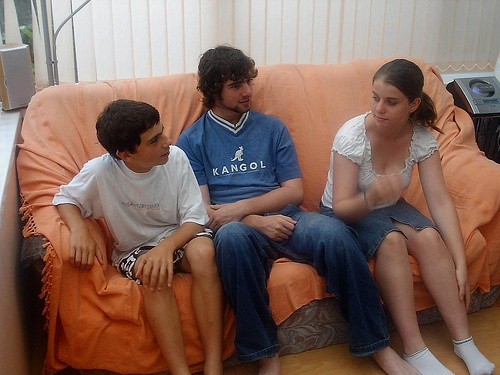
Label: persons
xmin=319 ymin=59 xmax=495 ymax=375
xmin=175 ymin=45 xmax=418 ymax=375
xmin=52 ymin=99 xmax=225 ymax=375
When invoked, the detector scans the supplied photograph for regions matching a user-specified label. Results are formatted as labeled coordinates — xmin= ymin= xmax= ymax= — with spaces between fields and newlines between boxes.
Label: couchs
xmin=16 ymin=56 xmax=500 ymax=375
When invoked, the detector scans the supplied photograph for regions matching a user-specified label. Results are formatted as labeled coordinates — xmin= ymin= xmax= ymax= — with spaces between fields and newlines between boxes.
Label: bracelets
xmin=363 ymin=189 xmax=368 ymax=208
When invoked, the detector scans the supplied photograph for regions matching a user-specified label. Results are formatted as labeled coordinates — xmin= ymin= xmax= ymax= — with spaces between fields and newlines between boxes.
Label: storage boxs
xmin=0 ymin=42 xmax=36 ymax=111
